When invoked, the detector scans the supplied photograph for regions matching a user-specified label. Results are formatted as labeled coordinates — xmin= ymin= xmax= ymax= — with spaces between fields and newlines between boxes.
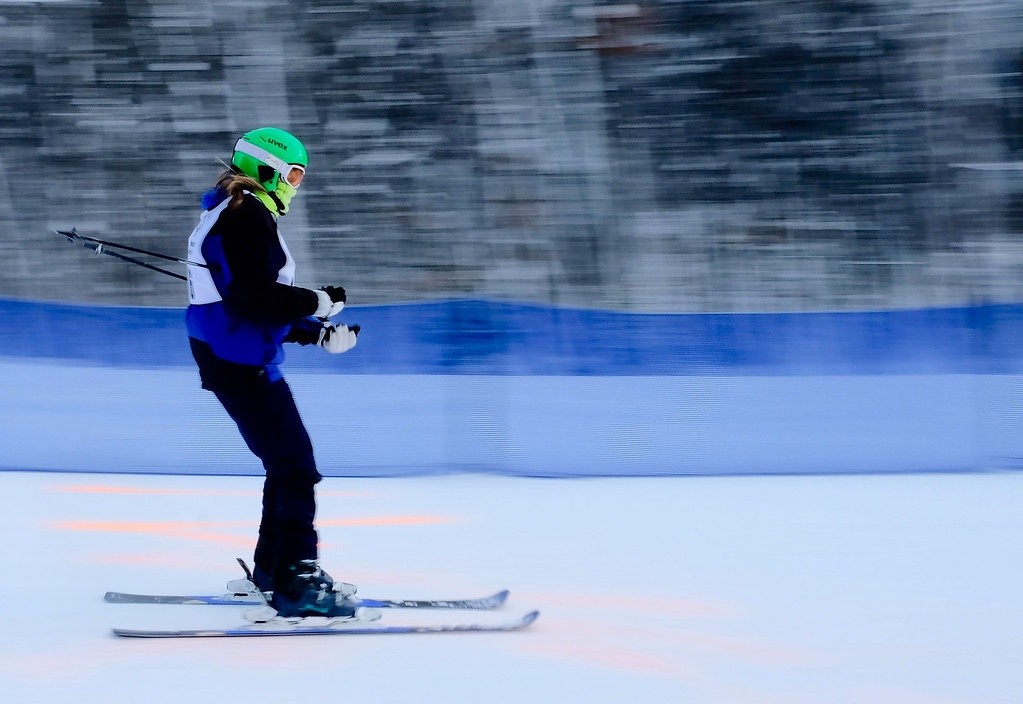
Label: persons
xmin=184 ymin=127 xmax=360 ymax=617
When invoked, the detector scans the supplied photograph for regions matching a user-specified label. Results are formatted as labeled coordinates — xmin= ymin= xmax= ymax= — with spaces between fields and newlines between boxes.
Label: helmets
xmin=230 ymin=127 xmax=307 ymax=218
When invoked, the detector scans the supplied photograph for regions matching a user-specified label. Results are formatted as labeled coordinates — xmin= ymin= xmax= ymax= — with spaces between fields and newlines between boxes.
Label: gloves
xmin=316 ymin=321 xmax=360 ymax=355
xmin=312 ymin=285 xmax=346 ymax=318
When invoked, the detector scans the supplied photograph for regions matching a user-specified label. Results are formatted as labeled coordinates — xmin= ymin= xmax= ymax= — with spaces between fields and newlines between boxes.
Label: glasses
xmin=281 ymin=164 xmax=305 ymax=189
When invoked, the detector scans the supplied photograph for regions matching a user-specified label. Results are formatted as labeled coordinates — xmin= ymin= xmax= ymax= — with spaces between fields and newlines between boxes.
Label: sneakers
xmin=254 ymin=560 xmax=332 ymax=598
xmin=270 ymin=569 xmax=358 ymax=618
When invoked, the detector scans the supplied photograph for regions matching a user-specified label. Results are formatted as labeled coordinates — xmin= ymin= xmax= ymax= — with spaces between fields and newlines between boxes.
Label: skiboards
xmin=104 ymin=589 xmax=540 ymax=639
